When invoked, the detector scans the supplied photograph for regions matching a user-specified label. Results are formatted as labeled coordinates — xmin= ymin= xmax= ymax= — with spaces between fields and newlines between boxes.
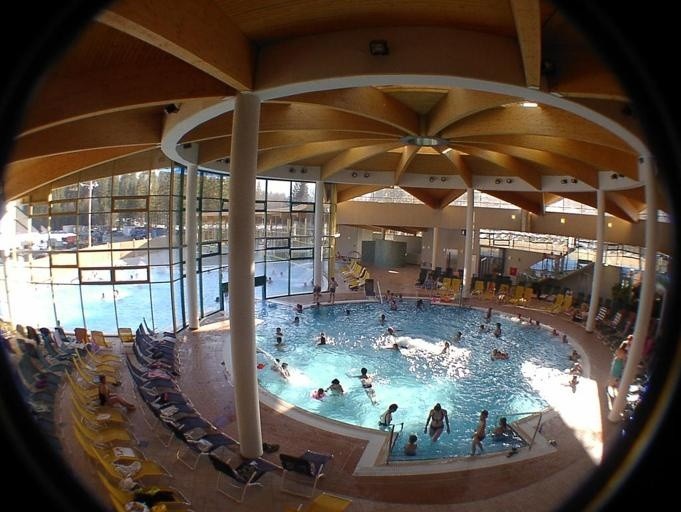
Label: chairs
xmin=276 ymin=449 xmax=334 ymax=500
xmin=209 ymin=454 xmax=287 ymax=503
xmin=340 ymin=260 xmax=369 ymax=290
xmin=297 ymin=492 xmax=351 ymax=511
xmin=1 ymin=318 xmax=241 ymax=511
xmin=414 ymin=267 xmax=638 ymax=348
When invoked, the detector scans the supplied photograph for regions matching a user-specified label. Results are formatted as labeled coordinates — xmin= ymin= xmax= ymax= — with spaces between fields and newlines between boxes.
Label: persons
xmin=479 ymin=324 xmax=486 ymax=332
xmin=329 ymin=276 xmax=339 ymax=305
xmin=536 ymin=321 xmax=541 ymax=328
xmin=379 ymin=403 xmax=399 ymax=430
xmin=552 ymin=329 xmax=557 ymax=335
xmin=518 ymin=313 xmax=522 ymax=319
xmin=528 ymin=317 xmax=533 ymax=325
xmin=318 ymin=332 xmax=327 ymax=345
xmin=326 ymin=378 xmax=345 ymax=396
xmin=469 ymin=410 xmax=489 ymax=457
xmin=495 ymin=416 xmax=507 ymax=432
xmin=562 ymin=333 xmax=569 ymax=343
xmin=298 ymin=305 xmax=303 ymax=311
xmin=295 ymin=304 xmax=300 ymax=310
xmin=98 ymin=374 xmax=137 ymax=412
xmin=567 ymin=348 xmax=583 ymax=384
xmin=281 ymin=362 xmax=290 ymax=378
xmin=416 ymin=298 xmax=424 ymax=310
xmin=275 ymin=327 xmax=283 ymax=344
xmin=492 ymin=349 xmax=507 ymax=361
xmin=485 ymin=307 xmax=493 ymax=320
xmin=456 ymin=332 xmax=463 ymax=340
xmin=424 ymin=403 xmax=451 ymax=441
xmin=384 ymin=290 xmax=403 ymax=310
xmin=393 ymin=343 xmax=400 ymax=350
xmin=385 ymin=327 xmax=397 ymax=338
xmin=271 ymin=358 xmax=280 ymax=371
xmin=380 ymin=314 xmax=388 ymax=322
xmin=494 ymin=322 xmax=502 ymax=336
xmin=346 ymin=367 xmax=378 ymax=405
xmin=295 ymin=317 xmax=299 ymax=322
xmin=312 ymin=388 xmax=326 ymax=399
xmin=404 ymin=435 xmax=418 ymax=457
xmin=443 ymin=342 xmax=450 ymax=353
xmin=608 ymin=333 xmax=634 ymax=386
